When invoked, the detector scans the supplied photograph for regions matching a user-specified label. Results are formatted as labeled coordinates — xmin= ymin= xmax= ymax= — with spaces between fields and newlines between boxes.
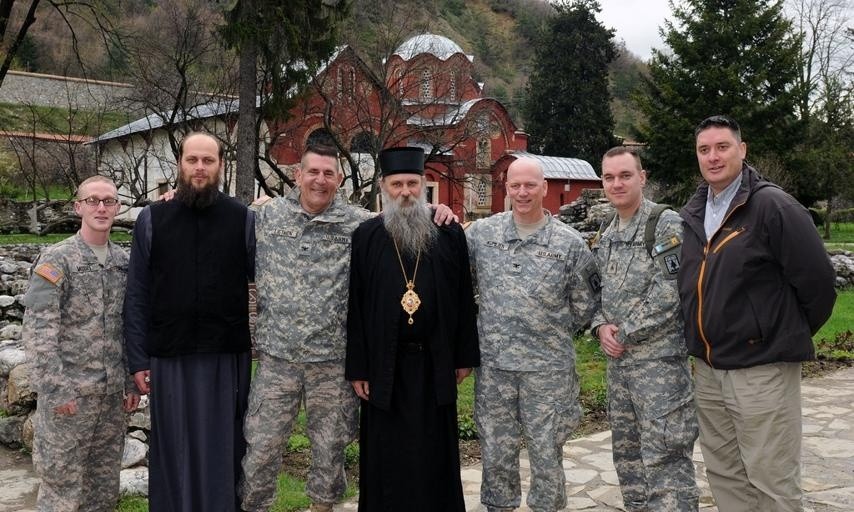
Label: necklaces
xmin=392 ymin=231 xmax=424 ymax=326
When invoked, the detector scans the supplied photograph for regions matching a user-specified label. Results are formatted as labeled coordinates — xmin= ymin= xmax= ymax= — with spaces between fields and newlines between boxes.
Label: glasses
xmin=79 ymin=196 xmax=119 ymax=207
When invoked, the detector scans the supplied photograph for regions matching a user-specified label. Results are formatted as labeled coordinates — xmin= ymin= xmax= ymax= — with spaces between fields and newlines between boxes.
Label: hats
xmin=379 ymin=146 xmax=424 ymax=177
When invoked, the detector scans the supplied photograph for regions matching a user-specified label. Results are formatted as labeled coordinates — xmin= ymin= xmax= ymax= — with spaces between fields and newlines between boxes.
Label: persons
xmin=122 ymin=133 xmax=257 ymax=512
xmin=344 ymin=147 xmax=480 ymax=512
xmin=679 ymin=115 xmax=837 ymax=512
xmin=157 ymin=142 xmax=459 ymax=512
xmin=22 ymin=175 xmax=143 ymax=511
xmin=589 ymin=146 xmax=701 ymax=512
xmin=463 ymin=157 xmax=601 ymax=511
